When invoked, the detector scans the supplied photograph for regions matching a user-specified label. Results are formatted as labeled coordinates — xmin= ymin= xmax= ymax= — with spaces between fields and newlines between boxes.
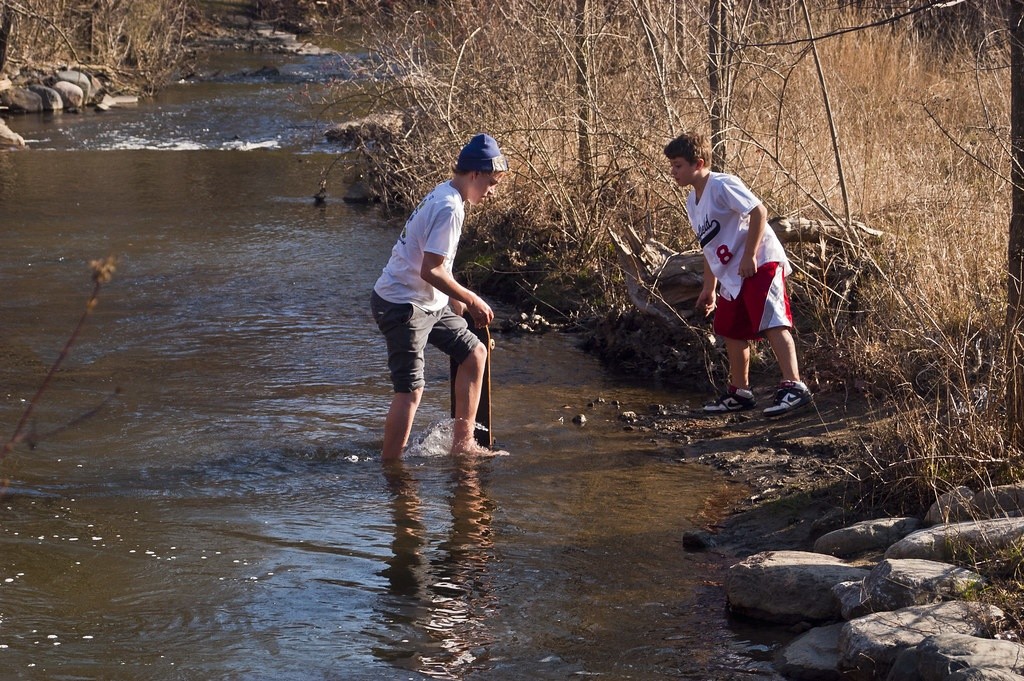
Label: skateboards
xmin=448 ymin=308 xmax=495 ymax=453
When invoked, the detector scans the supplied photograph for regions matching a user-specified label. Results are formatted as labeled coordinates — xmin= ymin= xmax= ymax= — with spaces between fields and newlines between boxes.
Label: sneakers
xmin=703 ymin=388 xmax=758 ymax=413
xmin=763 ymin=381 xmax=812 ymax=420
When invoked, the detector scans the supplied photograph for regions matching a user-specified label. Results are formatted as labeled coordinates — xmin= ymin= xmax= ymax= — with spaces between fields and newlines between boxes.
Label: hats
xmin=458 ymin=134 xmax=510 ymax=172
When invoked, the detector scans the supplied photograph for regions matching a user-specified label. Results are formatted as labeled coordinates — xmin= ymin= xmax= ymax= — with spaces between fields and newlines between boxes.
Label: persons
xmin=369 ymin=134 xmax=509 ymax=463
xmin=663 ymin=131 xmax=813 ymax=417
